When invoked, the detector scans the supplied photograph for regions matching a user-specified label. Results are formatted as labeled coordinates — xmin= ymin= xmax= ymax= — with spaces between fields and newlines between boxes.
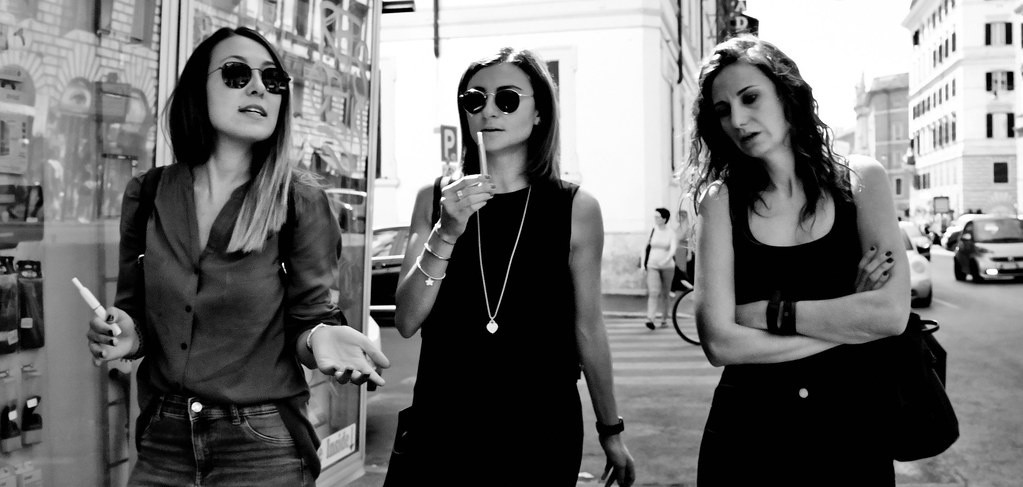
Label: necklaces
xmin=476 ymin=184 xmax=531 ymax=335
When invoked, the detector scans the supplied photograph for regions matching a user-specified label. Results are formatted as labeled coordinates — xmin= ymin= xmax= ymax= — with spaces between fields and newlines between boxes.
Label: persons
xmin=87 ymin=26 xmax=389 ymax=487
xmin=672 ymin=33 xmax=913 ymax=487
xmin=394 ymin=47 xmax=637 ymax=487
xmin=642 ymin=207 xmax=676 ymax=330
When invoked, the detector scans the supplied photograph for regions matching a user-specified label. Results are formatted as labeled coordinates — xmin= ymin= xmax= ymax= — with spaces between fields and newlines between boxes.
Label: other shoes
xmin=660 ymin=322 xmax=668 ymax=327
xmin=645 ymin=321 xmax=655 ymax=330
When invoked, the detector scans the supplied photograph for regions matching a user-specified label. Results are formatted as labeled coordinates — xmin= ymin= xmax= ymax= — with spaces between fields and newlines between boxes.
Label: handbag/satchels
xmin=639 ymin=228 xmax=654 ymax=270
xmin=835 ymin=311 xmax=960 ymax=464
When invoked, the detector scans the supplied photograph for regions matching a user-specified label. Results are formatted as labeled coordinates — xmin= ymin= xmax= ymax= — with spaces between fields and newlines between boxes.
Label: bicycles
xmin=671 ymin=288 xmax=702 ymax=346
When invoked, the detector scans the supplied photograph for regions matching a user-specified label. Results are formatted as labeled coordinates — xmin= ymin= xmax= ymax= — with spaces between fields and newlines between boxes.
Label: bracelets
xmin=434 ymin=223 xmax=456 ymax=246
xmin=424 ymin=243 xmax=453 ymax=263
xmin=766 ymin=290 xmax=783 ymax=336
xmin=595 ymin=415 xmax=626 ymax=436
xmin=305 ymin=323 xmax=330 ymax=354
xmin=416 ymin=256 xmax=446 ymax=286
xmin=783 ymin=300 xmax=797 ymax=336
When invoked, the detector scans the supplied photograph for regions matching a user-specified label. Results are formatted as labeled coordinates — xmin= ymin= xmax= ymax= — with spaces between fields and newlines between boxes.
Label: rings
xmin=457 ymin=190 xmax=465 ymax=201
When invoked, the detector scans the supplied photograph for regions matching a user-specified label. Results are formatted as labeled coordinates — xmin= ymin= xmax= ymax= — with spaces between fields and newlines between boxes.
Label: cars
xmin=368 ymin=221 xmax=411 ymax=329
xmin=898 ymin=211 xmax=1023 ymax=309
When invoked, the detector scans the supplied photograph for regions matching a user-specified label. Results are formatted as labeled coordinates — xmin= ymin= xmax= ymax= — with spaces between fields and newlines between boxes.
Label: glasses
xmin=206 ymin=62 xmax=291 ymax=94
xmin=458 ymin=89 xmax=536 ymax=116
